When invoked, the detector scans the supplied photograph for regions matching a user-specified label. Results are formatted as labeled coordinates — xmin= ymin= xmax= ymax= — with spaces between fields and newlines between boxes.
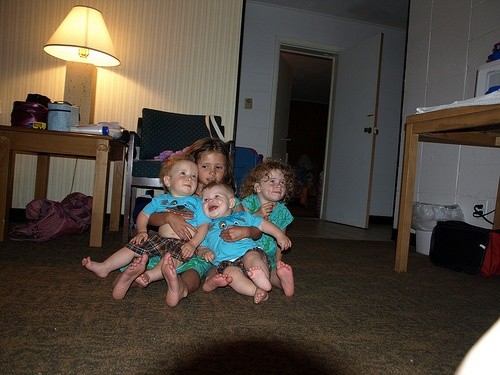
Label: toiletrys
xmin=70 ymin=126 xmax=110 ymax=135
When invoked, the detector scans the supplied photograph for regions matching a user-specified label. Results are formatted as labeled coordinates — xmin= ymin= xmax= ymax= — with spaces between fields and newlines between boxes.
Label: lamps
xmin=41 ymin=5 xmax=122 ymax=127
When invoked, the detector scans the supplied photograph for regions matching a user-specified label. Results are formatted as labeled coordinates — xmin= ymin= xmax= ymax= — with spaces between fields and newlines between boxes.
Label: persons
xmin=203 ymin=159 xmax=297 ymax=297
xmin=181 ymin=182 xmax=292 ymax=304
xmin=83 ymin=153 xmax=211 ymax=288
xmin=113 ymin=136 xmax=261 ymax=308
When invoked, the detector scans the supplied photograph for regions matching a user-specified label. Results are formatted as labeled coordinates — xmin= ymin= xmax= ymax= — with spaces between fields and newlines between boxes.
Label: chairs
xmin=235 ymin=146 xmax=264 ymax=199
xmin=121 ymin=106 xmax=225 ymax=244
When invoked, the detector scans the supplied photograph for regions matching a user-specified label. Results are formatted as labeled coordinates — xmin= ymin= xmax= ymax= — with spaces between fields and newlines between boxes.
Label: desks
xmin=393 ymin=102 xmax=500 ymax=273
xmin=0 ymin=125 xmax=127 ymax=248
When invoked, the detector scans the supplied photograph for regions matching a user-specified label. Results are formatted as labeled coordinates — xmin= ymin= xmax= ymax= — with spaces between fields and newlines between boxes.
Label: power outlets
xmin=477 ymin=200 xmax=488 ymax=221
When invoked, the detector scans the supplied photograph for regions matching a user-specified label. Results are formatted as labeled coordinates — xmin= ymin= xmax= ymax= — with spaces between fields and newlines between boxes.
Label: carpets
xmin=0 ymin=234 xmax=500 ymax=375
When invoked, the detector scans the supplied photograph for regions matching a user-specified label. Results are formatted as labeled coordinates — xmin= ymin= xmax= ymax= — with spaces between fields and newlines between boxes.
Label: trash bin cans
xmin=413 ymin=202 xmax=461 ymax=256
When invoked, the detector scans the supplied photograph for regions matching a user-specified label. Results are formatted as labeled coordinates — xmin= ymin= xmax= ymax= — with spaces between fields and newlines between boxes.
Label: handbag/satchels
xmin=429 ymin=220 xmax=500 ymax=279
xmin=8 ymin=192 xmax=93 ymax=242
xmin=205 ymin=112 xmax=235 ymax=184
